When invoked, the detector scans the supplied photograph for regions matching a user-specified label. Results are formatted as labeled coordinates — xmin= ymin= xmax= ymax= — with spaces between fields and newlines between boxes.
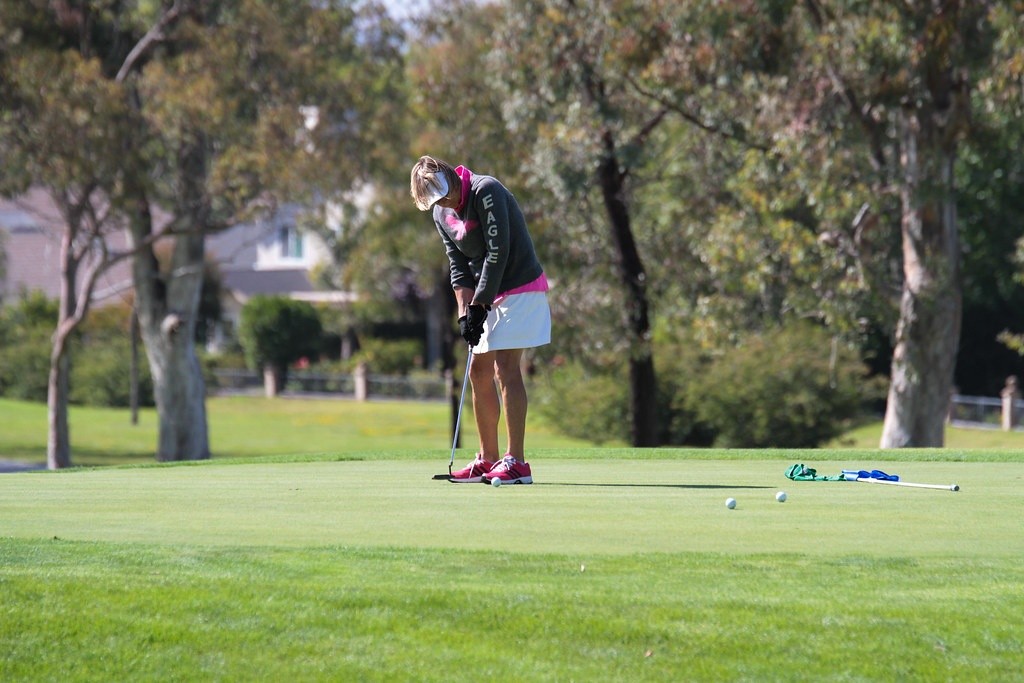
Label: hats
xmin=416 ymin=170 xmax=449 ymax=211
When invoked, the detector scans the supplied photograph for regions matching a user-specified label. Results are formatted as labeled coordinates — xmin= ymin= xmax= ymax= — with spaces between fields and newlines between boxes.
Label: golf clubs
xmin=430 ymin=342 xmax=474 ymax=480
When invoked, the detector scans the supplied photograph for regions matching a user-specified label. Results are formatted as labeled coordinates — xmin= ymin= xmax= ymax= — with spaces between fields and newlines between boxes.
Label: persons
xmin=411 ymin=155 xmax=551 ymax=485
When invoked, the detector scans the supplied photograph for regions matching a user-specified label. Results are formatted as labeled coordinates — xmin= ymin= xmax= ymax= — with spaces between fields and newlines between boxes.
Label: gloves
xmin=465 ymin=300 xmax=491 ymax=340
xmin=458 ymin=315 xmax=481 ymax=348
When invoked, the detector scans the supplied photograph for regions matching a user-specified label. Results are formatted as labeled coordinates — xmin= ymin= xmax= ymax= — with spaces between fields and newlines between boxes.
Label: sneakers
xmin=481 ymin=454 xmax=532 ymax=485
xmin=448 ymin=453 xmax=502 ymax=482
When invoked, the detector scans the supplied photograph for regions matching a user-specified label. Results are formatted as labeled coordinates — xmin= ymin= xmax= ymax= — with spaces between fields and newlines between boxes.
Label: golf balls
xmin=491 ymin=477 xmax=503 ymax=488
xmin=725 ymin=498 xmax=737 ymax=509
xmin=776 ymin=492 xmax=787 ymax=502
